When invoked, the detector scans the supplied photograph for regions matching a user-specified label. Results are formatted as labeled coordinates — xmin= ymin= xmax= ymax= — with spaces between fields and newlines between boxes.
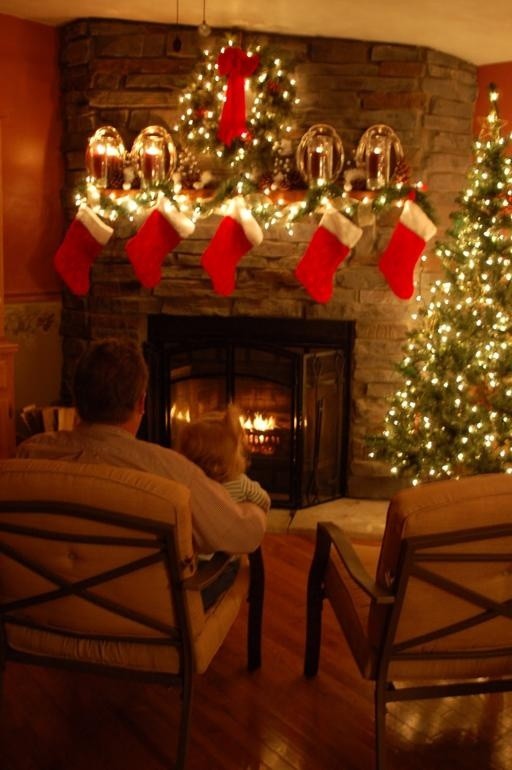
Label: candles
xmin=93 ymin=144 xmax=105 ymax=180
xmin=370 ymin=147 xmax=385 ymax=177
xmin=143 ymin=143 xmax=163 ymax=178
xmin=312 ymin=146 xmax=328 ymax=178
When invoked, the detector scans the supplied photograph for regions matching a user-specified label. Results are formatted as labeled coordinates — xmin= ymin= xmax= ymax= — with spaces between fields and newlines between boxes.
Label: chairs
xmin=303 ymin=523 xmax=511 ymax=770
xmin=1 ymin=500 xmax=265 ymax=770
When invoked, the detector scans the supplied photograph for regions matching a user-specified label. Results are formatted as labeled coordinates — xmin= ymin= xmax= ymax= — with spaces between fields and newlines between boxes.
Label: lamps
xmin=198 ymin=1 xmax=212 ymax=37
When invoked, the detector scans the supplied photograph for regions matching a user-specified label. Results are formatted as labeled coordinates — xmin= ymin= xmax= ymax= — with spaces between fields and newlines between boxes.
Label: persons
xmin=11 ymin=337 xmax=266 ymax=612
xmin=179 ymin=413 xmax=272 ymax=571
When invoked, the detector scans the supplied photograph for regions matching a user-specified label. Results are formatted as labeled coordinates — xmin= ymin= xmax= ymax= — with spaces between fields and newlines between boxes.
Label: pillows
xmin=0 ymin=457 xmax=207 ymax=642
xmin=366 ymin=474 xmax=511 ymax=652
